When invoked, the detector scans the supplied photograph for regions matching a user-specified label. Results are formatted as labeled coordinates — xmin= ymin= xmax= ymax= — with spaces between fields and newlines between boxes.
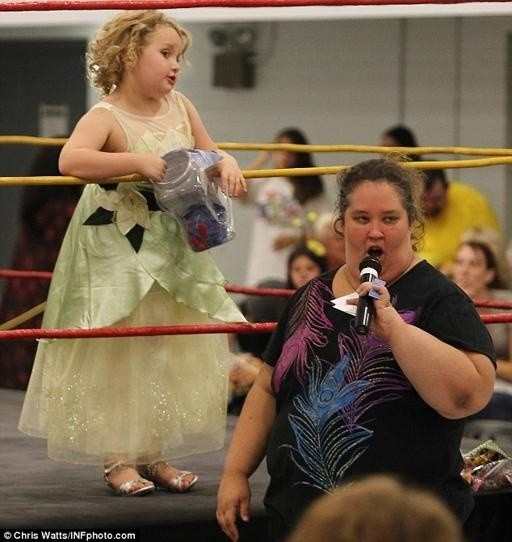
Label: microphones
xmin=354 ymin=254 xmax=383 ymax=335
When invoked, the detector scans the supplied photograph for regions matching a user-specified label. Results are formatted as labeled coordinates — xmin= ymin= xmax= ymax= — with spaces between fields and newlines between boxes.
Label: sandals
xmin=103 ymin=462 xmax=156 ymax=497
xmin=138 ymin=458 xmax=199 ymax=493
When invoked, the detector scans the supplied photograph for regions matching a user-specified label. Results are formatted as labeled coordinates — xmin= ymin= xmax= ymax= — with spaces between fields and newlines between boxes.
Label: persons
xmin=14 ymin=10 xmax=253 ymax=499
xmin=214 ymin=149 xmax=500 ymax=540
xmin=284 ymin=471 xmax=465 ymax=541
xmin=1 ymin=124 xmax=511 ymax=419
